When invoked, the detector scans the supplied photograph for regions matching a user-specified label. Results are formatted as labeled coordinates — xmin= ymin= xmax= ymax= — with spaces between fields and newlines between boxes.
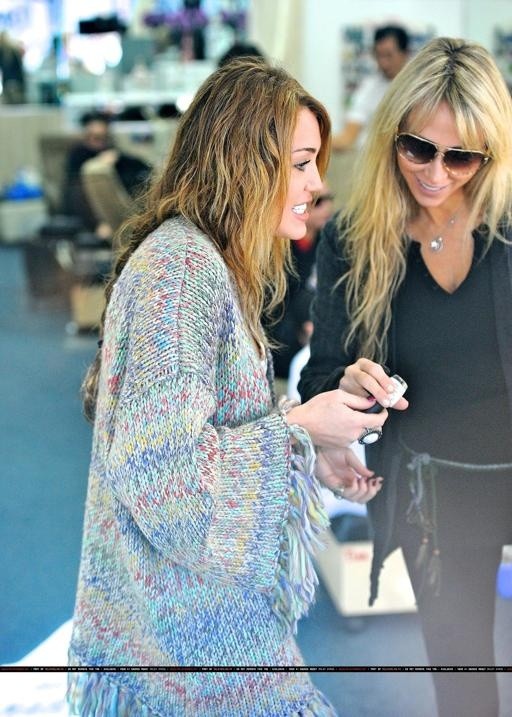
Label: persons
xmin=4 ymin=78 xmax=30 ymax=102
xmin=64 ymin=50 xmax=393 ymax=716
xmin=257 ymin=177 xmax=336 ymax=382
xmin=328 ymin=21 xmax=413 ymax=156
xmin=39 ymin=36 xmax=59 ymax=71
xmin=1 ymin=31 xmax=28 ymax=94
xmin=62 ymin=103 xmax=186 ymax=249
xmin=216 ymin=39 xmax=263 ymax=67
xmin=294 ymin=35 xmax=512 ymax=716
xmin=38 ymin=82 xmax=59 ymax=104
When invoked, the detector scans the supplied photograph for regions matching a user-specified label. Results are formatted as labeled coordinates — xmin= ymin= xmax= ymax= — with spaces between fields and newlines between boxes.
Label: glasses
xmin=393 ymin=129 xmax=492 ymax=178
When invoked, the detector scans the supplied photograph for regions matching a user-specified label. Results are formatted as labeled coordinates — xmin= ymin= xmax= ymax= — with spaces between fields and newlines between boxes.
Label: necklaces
xmin=412 ymin=198 xmax=470 ymax=253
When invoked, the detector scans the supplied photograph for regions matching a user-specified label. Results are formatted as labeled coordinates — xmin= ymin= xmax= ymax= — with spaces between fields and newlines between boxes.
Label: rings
xmin=357 ymin=424 xmax=382 ymax=446
xmin=332 ymin=485 xmax=345 ymax=499
